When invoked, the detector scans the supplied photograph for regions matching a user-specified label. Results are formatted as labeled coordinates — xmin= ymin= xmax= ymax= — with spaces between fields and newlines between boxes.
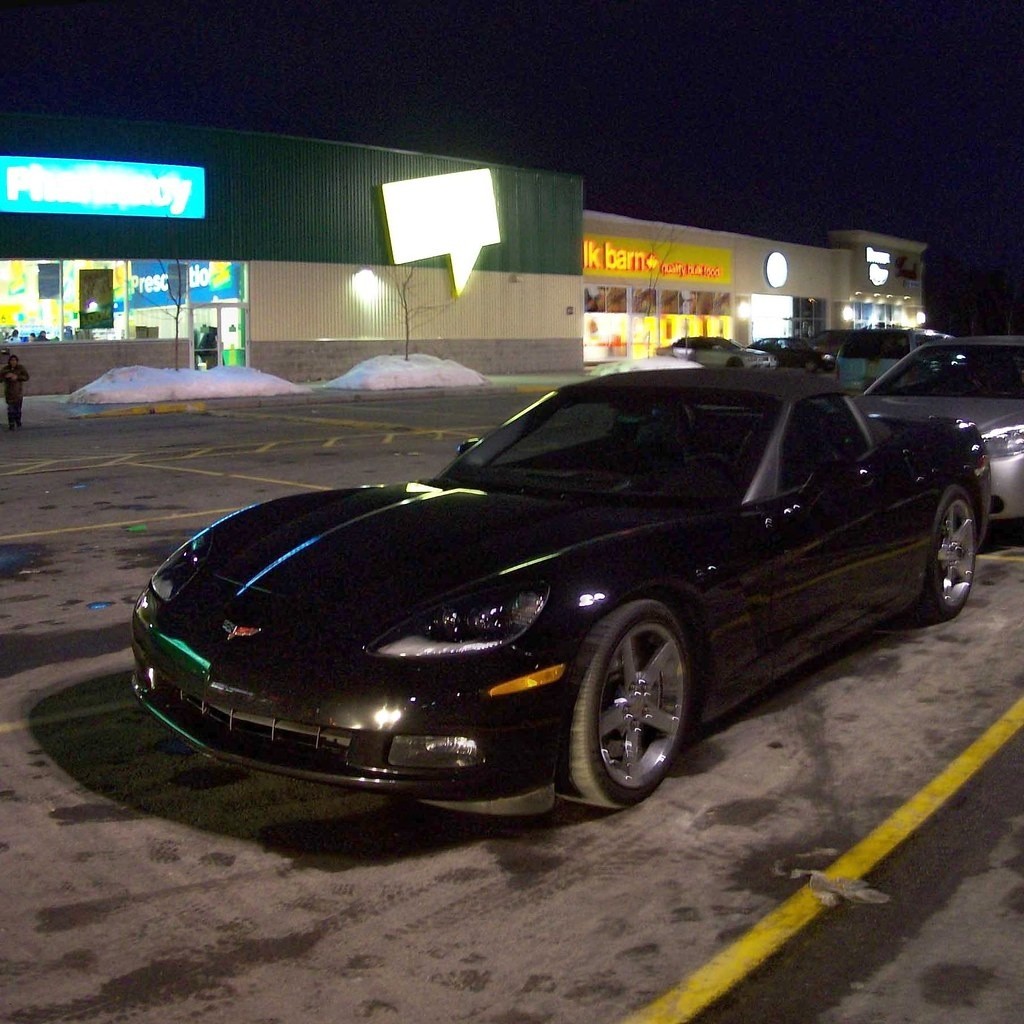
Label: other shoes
xmin=9 ymin=421 xmax=15 ymax=430
xmin=16 ymin=419 xmax=21 ymax=428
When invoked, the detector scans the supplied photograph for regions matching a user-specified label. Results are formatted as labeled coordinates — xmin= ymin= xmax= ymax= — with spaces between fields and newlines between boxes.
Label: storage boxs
xmin=129 ymin=325 xmax=147 ymax=338
xmin=148 ymin=326 xmax=159 ymax=338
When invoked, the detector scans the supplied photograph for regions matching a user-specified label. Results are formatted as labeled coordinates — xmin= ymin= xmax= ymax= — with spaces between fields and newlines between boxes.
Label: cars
xmin=655 ymin=327 xmax=1024 ymax=522
xmin=132 ymin=368 xmax=989 ymax=819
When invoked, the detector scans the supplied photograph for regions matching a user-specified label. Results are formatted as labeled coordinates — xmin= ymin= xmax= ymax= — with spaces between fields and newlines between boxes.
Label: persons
xmin=0 ymin=354 xmax=30 ymax=431
xmin=0 ymin=330 xmax=51 ymax=344
xmin=198 ymin=327 xmax=219 ymax=369
